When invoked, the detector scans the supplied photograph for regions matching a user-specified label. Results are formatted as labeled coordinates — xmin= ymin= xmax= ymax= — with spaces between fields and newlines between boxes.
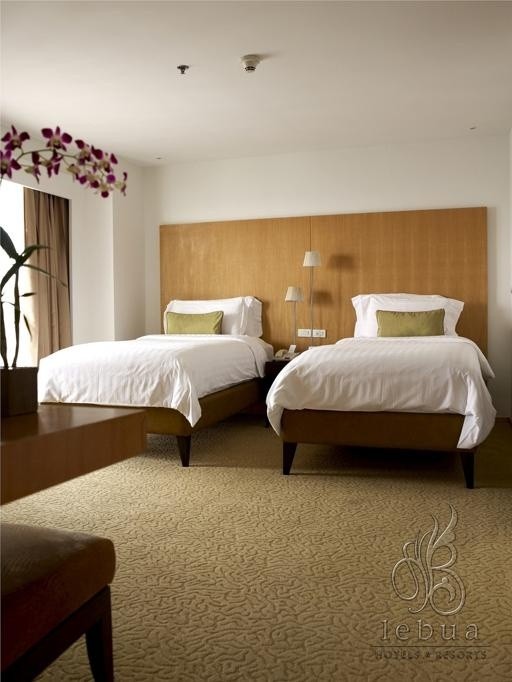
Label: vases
xmin=1 ymin=367 xmax=37 ymax=417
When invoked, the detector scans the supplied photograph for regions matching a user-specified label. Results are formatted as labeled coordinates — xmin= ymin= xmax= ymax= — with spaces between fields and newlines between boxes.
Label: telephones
xmin=275 ymin=345 xmax=299 ymax=361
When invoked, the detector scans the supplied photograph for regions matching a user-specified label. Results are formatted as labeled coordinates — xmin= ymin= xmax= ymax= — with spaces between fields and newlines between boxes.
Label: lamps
xmin=284 ymin=250 xmax=323 ymax=357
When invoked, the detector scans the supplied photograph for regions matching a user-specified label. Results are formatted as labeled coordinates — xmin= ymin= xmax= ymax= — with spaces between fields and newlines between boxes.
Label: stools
xmin=1 ymin=522 xmax=117 ymax=682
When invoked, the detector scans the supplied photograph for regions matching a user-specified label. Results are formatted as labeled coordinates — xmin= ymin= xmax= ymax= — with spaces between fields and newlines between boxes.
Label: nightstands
xmin=263 ymin=359 xmax=297 ymax=429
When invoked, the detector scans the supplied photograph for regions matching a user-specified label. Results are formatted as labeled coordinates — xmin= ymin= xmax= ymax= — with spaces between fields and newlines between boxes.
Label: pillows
xmin=164 ymin=309 xmax=223 ymax=335
xmin=376 ymin=307 xmax=445 ymax=338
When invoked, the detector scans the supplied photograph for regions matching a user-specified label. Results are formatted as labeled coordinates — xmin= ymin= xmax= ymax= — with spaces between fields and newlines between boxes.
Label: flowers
xmin=0 ymin=123 xmax=129 ymax=368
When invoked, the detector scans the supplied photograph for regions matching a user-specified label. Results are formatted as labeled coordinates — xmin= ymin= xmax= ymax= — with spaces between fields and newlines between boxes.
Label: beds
xmin=265 ymin=291 xmax=490 ymax=489
xmin=37 ymin=294 xmax=273 ymax=466
